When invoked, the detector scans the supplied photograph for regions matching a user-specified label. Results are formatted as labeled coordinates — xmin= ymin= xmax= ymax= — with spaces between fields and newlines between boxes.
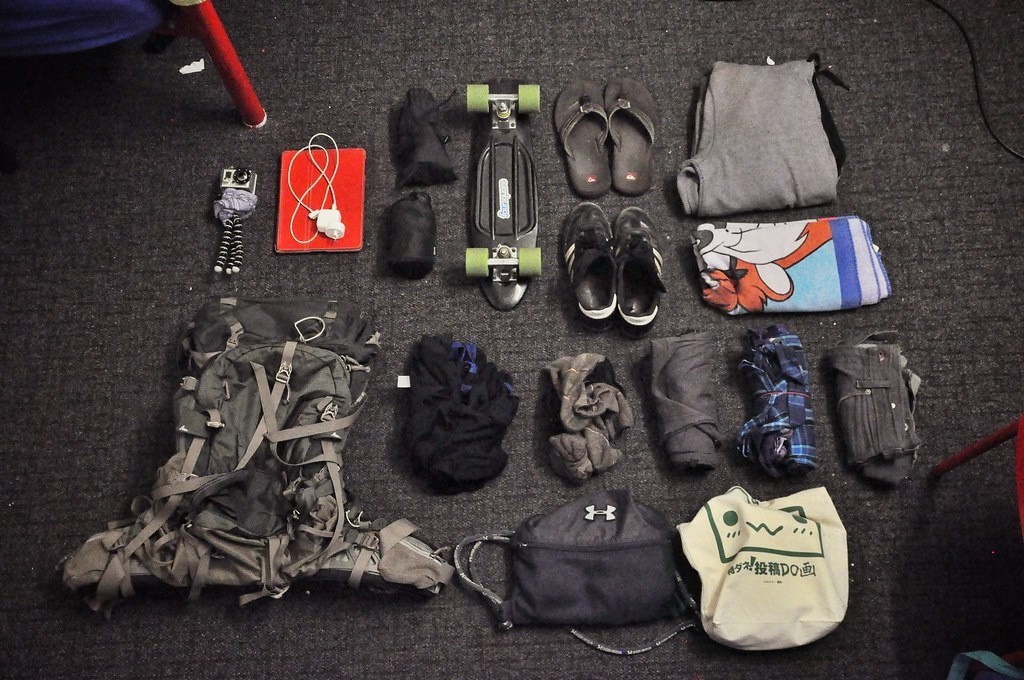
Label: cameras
xmin=219 ymin=169 xmax=257 ymax=195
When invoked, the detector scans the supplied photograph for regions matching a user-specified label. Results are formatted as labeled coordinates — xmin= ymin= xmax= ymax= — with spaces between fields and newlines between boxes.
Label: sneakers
xmin=562 ymin=202 xmax=665 ymax=339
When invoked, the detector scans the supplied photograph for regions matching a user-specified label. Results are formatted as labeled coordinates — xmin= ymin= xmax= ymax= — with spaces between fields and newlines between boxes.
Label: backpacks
xmin=60 ymin=296 xmax=456 ymax=598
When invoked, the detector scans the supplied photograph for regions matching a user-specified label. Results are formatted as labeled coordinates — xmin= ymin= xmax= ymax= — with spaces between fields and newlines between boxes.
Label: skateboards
xmin=460 ymin=72 xmax=547 ymax=311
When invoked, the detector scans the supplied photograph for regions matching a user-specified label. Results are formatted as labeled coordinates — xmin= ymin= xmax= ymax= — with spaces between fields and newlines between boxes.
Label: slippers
xmin=554 ymin=78 xmax=657 ymax=196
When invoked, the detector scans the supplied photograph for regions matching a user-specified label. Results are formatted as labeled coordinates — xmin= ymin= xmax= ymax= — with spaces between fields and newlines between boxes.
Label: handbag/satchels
xmin=506 ymin=490 xmax=681 ymax=627
xmin=678 ymin=485 xmax=849 ymax=651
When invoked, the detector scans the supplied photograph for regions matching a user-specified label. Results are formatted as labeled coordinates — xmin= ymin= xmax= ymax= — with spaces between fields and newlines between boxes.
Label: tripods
xmin=214 ymin=213 xmax=243 ymax=276
